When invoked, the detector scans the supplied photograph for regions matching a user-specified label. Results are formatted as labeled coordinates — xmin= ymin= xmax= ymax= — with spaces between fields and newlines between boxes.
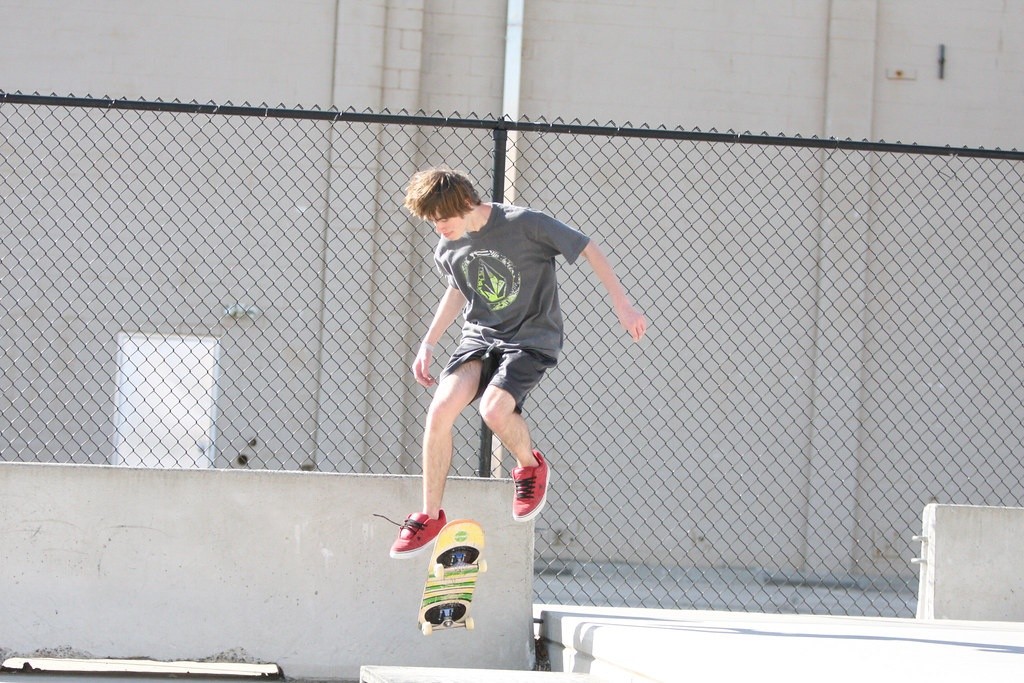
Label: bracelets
xmin=419 ymin=342 xmax=435 ymax=354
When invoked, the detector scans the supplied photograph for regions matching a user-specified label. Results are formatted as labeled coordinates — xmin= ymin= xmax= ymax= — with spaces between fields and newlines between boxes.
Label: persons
xmin=388 ymin=170 xmax=647 ymax=558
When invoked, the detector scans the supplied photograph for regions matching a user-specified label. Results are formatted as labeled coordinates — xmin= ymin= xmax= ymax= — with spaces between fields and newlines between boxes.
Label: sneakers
xmin=512 ymin=450 xmax=550 ymax=522
xmin=373 ymin=509 xmax=446 ymax=559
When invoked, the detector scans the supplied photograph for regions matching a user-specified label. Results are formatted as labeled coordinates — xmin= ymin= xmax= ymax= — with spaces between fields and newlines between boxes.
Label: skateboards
xmin=417 ymin=520 xmax=490 ymax=637
